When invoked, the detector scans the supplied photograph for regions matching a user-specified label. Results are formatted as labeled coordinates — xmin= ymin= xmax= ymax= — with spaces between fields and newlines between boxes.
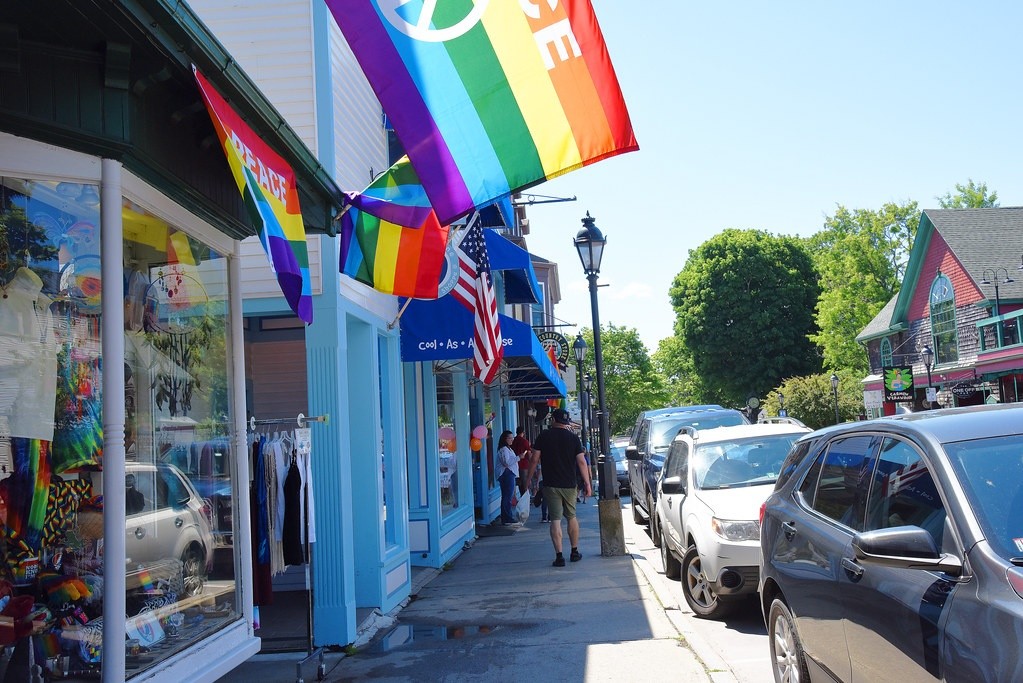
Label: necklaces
xmin=33 ymin=301 xmax=48 ymax=344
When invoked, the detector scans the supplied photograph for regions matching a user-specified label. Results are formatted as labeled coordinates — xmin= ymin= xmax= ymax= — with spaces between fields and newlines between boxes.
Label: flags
xmin=547 ymin=346 xmax=566 ymax=408
xmin=323 ymin=0 xmax=640 ymax=228
xmin=164 ymin=230 xmax=211 ymax=312
xmin=191 ymin=64 xmax=504 ymax=386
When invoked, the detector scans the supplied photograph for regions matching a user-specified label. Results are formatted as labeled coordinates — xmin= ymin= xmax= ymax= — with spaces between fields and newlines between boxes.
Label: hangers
xmin=155 ymin=419 xmax=313 ymax=454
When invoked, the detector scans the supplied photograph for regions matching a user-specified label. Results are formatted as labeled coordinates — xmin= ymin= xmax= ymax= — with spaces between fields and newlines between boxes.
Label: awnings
xmin=482 ymin=229 xmax=566 ymax=400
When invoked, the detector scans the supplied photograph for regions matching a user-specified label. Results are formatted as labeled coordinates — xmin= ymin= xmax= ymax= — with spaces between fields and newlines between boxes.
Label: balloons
xmin=471 ymin=426 xmax=487 ymax=452
xmin=438 ymin=429 xmax=456 ymax=452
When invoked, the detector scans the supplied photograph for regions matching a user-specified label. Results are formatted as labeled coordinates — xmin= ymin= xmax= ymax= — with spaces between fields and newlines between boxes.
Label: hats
xmin=552 ymin=410 xmax=570 ymax=424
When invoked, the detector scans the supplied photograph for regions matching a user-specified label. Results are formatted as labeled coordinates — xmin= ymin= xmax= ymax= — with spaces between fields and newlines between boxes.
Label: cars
xmin=60 ymin=433 xmax=262 ymax=618
xmin=610 ymin=436 xmax=633 ymax=495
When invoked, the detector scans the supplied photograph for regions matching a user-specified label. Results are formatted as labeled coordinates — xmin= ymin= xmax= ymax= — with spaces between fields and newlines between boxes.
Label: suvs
xmin=625 ymin=403 xmax=767 ymax=549
xmin=653 ymin=415 xmax=817 ymax=621
xmin=756 ymin=402 xmax=1023 ymax=683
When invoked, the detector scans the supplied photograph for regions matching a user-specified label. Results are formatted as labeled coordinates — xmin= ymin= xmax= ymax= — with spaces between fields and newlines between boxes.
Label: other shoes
xmin=505 ymin=520 xmax=520 ymax=525
xmin=543 ymin=520 xmax=547 ymax=522
xmin=549 ymin=520 xmax=552 ymax=522
xmin=583 ymin=502 xmax=586 ymax=504
xmin=577 ymin=498 xmax=581 ymax=502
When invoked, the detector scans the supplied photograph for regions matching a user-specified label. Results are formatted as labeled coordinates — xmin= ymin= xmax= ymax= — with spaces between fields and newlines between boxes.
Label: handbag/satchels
xmin=512 ymin=497 xmax=516 ymax=505
xmin=533 ymin=487 xmax=544 ymax=507
xmin=516 ymin=486 xmax=521 ymax=501
xmin=516 ymin=490 xmax=530 ymax=525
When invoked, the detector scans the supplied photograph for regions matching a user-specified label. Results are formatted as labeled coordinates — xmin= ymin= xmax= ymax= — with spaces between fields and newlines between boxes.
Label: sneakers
xmin=553 ymin=557 xmax=565 ymax=566
xmin=571 ymin=553 xmax=582 ymax=561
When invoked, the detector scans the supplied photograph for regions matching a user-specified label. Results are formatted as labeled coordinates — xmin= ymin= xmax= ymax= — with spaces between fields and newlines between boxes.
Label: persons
xmin=0 ymin=267 xmax=58 ymax=532
xmin=494 ymin=408 xmax=592 ymax=568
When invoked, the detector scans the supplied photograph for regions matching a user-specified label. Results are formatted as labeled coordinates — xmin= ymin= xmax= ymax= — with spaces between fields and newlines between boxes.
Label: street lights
xmin=582 ymin=371 xmax=600 ymax=465
xmin=778 ymin=392 xmax=784 ymax=417
xmin=920 ymin=343 xmax=934 ymax=409
xmin=830 ymin=373 xmax=840 ymax=424
xmin=572 ymin=209 xmax=620 ymax=501
xmin=979 ymin=267 xmax=1015 ymax=404
xmin=571 ymin=331 xmax=592 ymax=478
